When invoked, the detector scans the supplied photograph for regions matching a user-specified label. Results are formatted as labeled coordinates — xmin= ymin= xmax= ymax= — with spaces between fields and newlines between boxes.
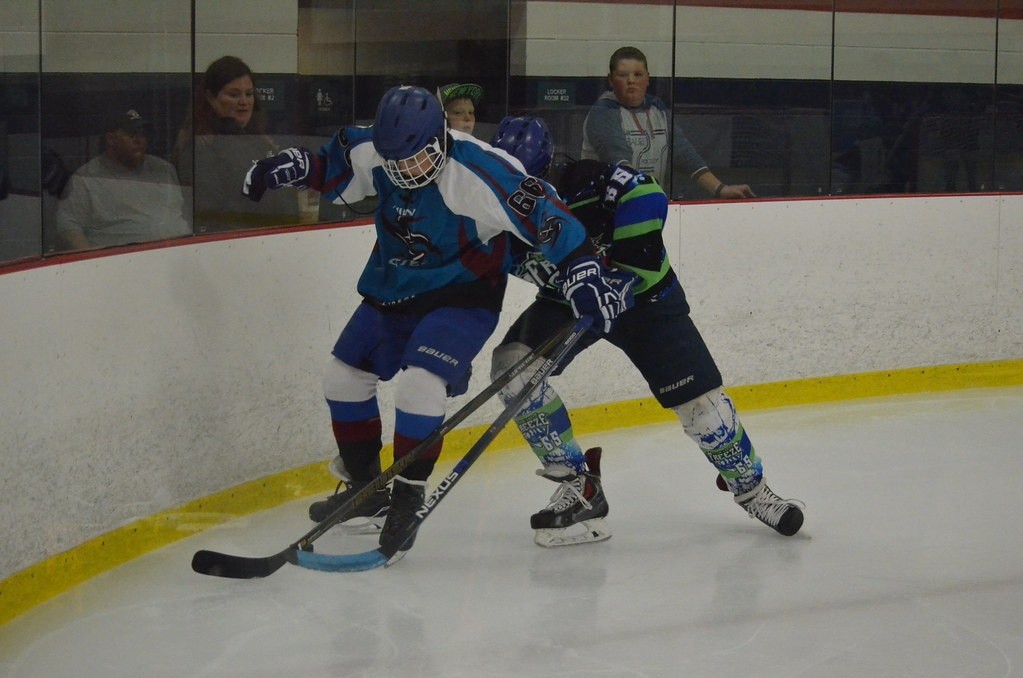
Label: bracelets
xmin=716 ymin=184 xmax=725 ymax=198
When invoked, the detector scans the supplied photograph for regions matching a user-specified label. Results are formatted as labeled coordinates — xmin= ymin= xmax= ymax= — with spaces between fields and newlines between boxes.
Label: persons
xmin=833 ymin=82 xmax=985 ymax=193
xmin=54 ymin=103 xmax=192 ymax=251
xmin=243 ymin=87 xmax=620 ymax=566
xmin=581 ymin=47 xmax=757 ymax=198
xmin=176 ymin=56 xmax=299 ymax=231
xmin=491 ymin=116 xmax=812 ymax=548
xmin=440 ymin=83 xmax=482 ymax=134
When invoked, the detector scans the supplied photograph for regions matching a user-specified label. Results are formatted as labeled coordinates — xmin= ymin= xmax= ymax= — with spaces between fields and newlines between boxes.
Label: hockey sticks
xmin=191 ymin=315 xmax=577 ymax=580
xmin=284 ymin=316 xmax=594 ymax=572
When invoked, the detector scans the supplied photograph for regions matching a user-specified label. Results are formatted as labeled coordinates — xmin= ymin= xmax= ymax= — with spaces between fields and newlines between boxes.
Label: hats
xmin=103 ymin=108 xmax=152 ymax=133
xmin=441 ymin=83 xmax=483 ymax=104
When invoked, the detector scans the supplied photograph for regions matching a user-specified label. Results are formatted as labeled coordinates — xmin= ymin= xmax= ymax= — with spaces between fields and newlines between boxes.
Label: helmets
xmin=489 ymin=115 xmax=554 ymax=179
xmin=372 ymin=85 xmax=448 ymax=190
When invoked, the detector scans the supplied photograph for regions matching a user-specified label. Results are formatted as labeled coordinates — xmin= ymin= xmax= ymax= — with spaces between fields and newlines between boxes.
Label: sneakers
xmin=308 ymin=455 xmax=391 ymax=533
xmin=379 ymin=475 xmax=429 ymax=567
xmin=716 ymin=474 xmax=804 ymax=536
xmin=530 ymin=447 xmax=612 ymax=546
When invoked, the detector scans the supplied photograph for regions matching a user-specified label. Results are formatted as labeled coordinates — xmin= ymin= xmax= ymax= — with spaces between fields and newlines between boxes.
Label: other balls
xmin=288 ymin=542 xmax=314 ymax=552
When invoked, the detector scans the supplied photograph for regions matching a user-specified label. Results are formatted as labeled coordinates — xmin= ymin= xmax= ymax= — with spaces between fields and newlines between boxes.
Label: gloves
xmin=603 ymin=269 xmax=641 ymax=312
xmin=562 ymin=256 xmax=622 ymax=338
xmin=242 ymin=145 xmax=312 ymax=202
